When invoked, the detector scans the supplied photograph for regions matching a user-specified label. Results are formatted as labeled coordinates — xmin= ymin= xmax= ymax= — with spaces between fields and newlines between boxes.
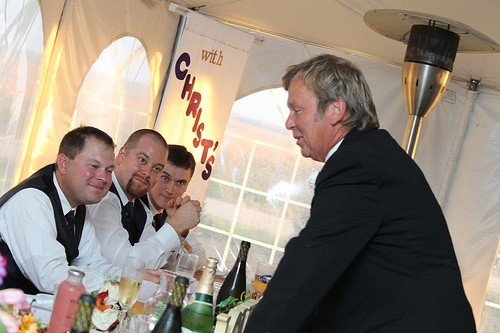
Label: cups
xmin=254 ymin=261 xmax=276 ymax=281
xmin=176 ymin=252 xmax=200 ymax=277
xmin=118 ymin=308 xmax=151 ymax=333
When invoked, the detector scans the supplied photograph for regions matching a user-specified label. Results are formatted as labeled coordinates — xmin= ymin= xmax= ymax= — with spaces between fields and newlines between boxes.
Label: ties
xmin=124 ymin=201 xmax=137 ymax=245
xmin=154 ymin=212 xmax=163 ymax=232
xmin=66 ymin=210 xmax=75 ymax=246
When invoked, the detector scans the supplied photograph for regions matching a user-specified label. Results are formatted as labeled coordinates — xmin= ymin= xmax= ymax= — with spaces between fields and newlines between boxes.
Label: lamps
xmin=363 ymin=8 xmax=500 ymax=160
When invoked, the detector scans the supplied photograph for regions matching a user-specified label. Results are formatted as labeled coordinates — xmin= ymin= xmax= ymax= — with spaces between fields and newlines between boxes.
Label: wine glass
xmin=116 ymin=257 xmax=145 ymax=333
xmin=142 ymin=268 xmax=198 ymax=333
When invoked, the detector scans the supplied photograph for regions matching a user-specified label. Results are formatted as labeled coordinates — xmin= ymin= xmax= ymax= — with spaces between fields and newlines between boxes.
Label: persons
xmin=0 ymin=125 xmax=162 ymax=296
xmin=137 ymin=144 xmax=196 ymax=232
xmin=244 ymin=52 xmax=477 ymax=333
xmin=87 ymin=130 xmax=202 ymax=267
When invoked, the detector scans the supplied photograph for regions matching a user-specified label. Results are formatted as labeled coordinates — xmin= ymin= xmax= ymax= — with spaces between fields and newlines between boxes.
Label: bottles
xmin=181 ymin=256 xmax=218 ymax=333
xmin=67 ymin=293 xmax=97 ymax=333
xmin=151 ymin=275 xmax=189 ymax=333
xmin=213 ymin=240 xmax=251 ymax=330
xmin=47 ymin=269 xmax=86 ymax=333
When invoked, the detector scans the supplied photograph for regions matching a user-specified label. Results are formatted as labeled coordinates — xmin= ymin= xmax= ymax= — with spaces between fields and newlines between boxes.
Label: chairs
xmin=213 ymin=293 xmax=265 ymax=333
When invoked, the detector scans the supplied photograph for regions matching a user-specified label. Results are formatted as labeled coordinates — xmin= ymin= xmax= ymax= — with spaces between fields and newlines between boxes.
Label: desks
xmin=0 ymin=266 xmax=256 ymax=333
